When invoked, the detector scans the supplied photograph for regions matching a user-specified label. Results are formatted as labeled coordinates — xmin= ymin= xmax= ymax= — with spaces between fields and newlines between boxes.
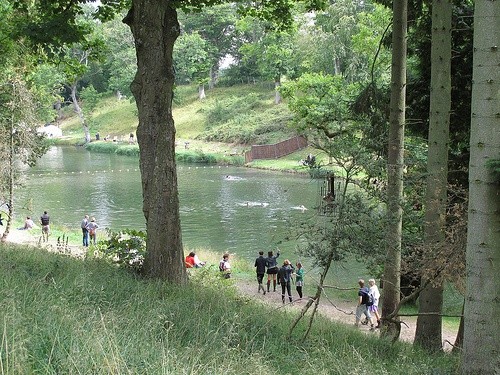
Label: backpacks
xmin=359 ymin=288 xmax=374 ymax=308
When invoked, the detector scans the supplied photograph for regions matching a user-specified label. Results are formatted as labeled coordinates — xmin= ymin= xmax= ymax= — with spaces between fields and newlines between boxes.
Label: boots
xmin=376 ymin=319 xmax=380 ymax=328
xmin=363 ymin=318 xmax=368 ymax=324
xmin=369 ymin=324 xmax=375 ymax=331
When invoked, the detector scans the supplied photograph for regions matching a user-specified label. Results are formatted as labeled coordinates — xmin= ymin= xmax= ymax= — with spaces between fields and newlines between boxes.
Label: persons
xmin=16 ymin=216 xmax=38 ymax=230
xmin=293 ymin=263 xmax=304 ymax=300
xmin=265 ymin=247 xmax=280 ymax=293
xmin=185 ymin=252 xmax=206 ymax=268
xmin=277 ymin=259 xmax=295 ymax=304
xmin=354 ymin=279 xmax=375 ymax=332
xmin=80 ymin=214 xmax=90 ymax=248
xmin=84 ymin=217 xmax=98 ymax=245
xmin=38 ymin=210 xmax=50 ymax=242
xmin=255 ymin=250 xmax=266 ymax=296
xmin=219 ymin=254 xmax=231 ymax=279
xmin=361 ymin=279 xmax=381 ymax=328
